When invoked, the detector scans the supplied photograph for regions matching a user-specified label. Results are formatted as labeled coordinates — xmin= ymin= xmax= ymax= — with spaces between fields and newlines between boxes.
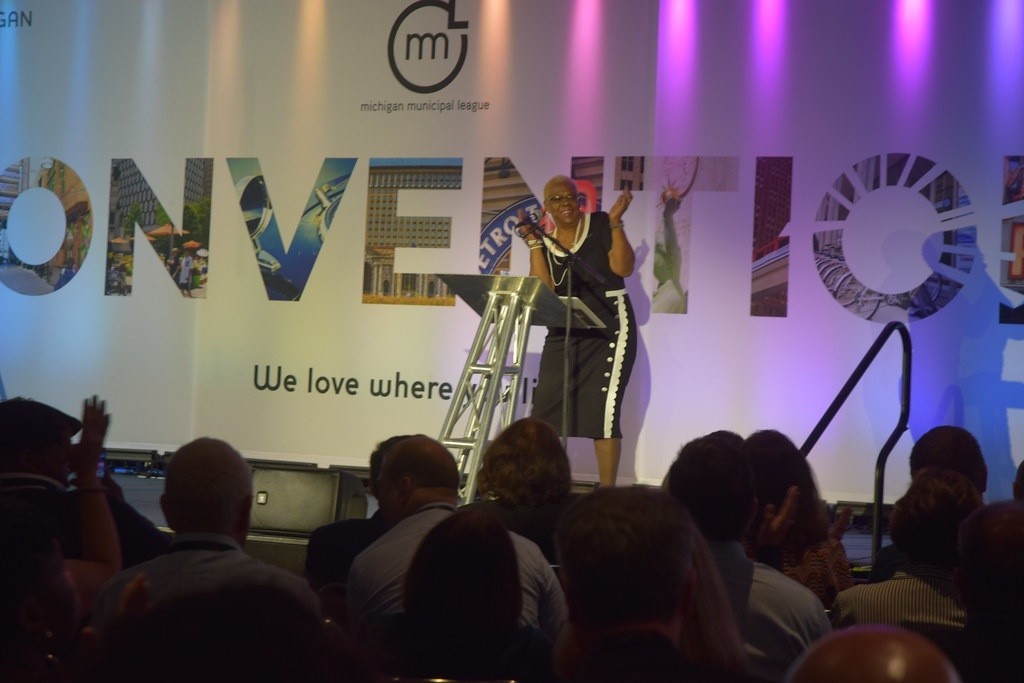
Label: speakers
xmin=243 ymin=460 xmax=371 ymax=540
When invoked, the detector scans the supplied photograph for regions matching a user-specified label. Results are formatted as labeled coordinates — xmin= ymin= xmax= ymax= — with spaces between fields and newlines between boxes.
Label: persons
xmin=518 ymin=175 xmax=637 ymax=487
xmin=0 ymin=396 xmax=1024 ymax=683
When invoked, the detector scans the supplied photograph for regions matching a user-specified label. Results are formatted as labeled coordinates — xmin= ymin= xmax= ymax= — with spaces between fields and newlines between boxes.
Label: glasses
xmin=547 ymin=194 xmax=577 ymax=204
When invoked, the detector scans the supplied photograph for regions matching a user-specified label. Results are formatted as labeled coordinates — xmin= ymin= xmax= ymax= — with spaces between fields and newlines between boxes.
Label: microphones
xmin=514 ymin=210 xmax=544 ymax=229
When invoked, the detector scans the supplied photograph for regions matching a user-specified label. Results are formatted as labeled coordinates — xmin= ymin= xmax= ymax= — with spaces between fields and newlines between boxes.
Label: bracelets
xmin=79 ymin=486 xmax=109 ymax=495
xmin=525 ymin=240 xmax=543 ymax=249
xmin=610 ymin=221 xmax=625 ymax=229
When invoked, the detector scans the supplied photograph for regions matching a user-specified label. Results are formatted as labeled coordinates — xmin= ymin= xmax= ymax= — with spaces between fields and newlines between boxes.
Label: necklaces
xmin=546 ymin=219 xmax=582 ymax=286
